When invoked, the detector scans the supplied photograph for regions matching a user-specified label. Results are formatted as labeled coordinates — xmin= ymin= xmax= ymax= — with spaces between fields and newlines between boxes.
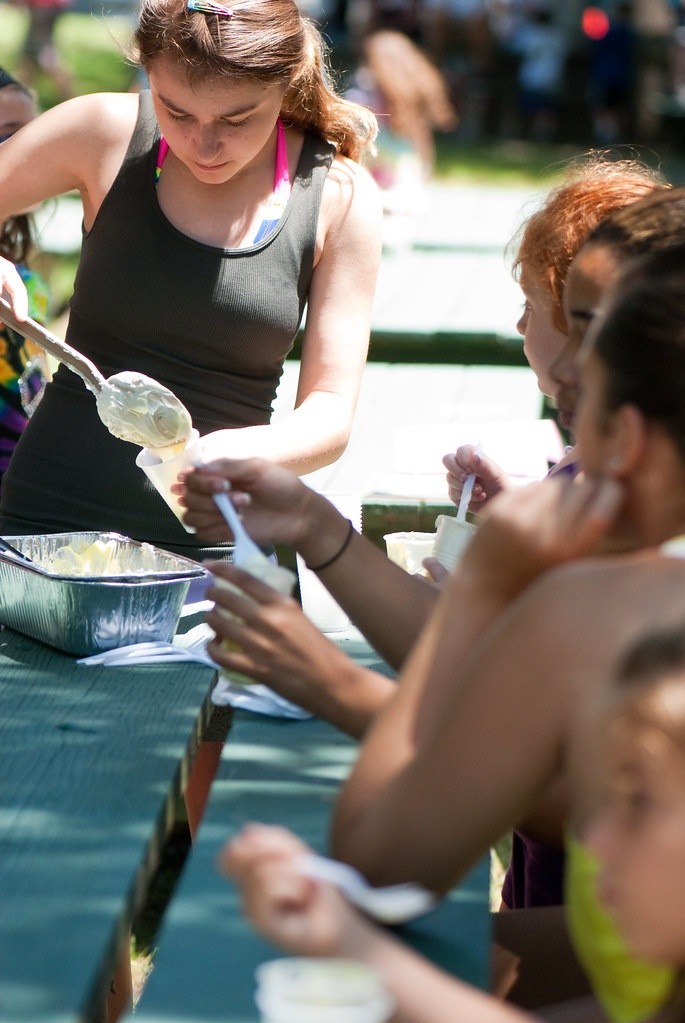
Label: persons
xmin=0 ymin=0 xmax=685 ymax=1023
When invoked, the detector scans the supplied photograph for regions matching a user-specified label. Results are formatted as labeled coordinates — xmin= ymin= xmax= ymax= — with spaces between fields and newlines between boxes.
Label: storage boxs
xmin=0 ymin=529 xmax=209 ymax=655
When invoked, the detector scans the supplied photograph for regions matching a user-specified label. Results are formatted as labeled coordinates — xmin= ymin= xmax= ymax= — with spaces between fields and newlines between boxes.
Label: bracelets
xmin=306 ymin=520 xmax=353 ymax=570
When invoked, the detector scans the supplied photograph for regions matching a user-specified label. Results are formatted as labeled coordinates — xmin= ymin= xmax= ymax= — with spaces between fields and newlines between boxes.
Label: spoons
xmin=191 ymin=456 xmax=269 ymax=575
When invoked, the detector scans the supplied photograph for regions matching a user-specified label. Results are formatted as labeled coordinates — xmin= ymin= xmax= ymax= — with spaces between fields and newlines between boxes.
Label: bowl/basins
xmin=0 ymin=530 xmax=209 ymax=657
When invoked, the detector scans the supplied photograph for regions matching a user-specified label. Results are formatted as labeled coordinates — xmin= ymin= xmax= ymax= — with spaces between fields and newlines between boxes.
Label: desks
xmin=0 ymin=598 xmax=512 ymax=1023
xmin=263 ymin=185 xmax=563 ymax=532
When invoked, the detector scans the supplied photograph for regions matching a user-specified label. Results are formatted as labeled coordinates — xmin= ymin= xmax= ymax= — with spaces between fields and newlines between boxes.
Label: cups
xmin=135 ymin=427 xmax=207 ymax=534
xmin=295 ymin=473 xmax=364 ymax=633
xmin=425 ymin=515 xmax=477 ymax=584
xmin=204 ymin=558 xmax=296 ymax=685
xmin=383 ymin=531 xmax=437 ymax=579
xmin=251 ymin=959 xmax=398 ymax=1023
